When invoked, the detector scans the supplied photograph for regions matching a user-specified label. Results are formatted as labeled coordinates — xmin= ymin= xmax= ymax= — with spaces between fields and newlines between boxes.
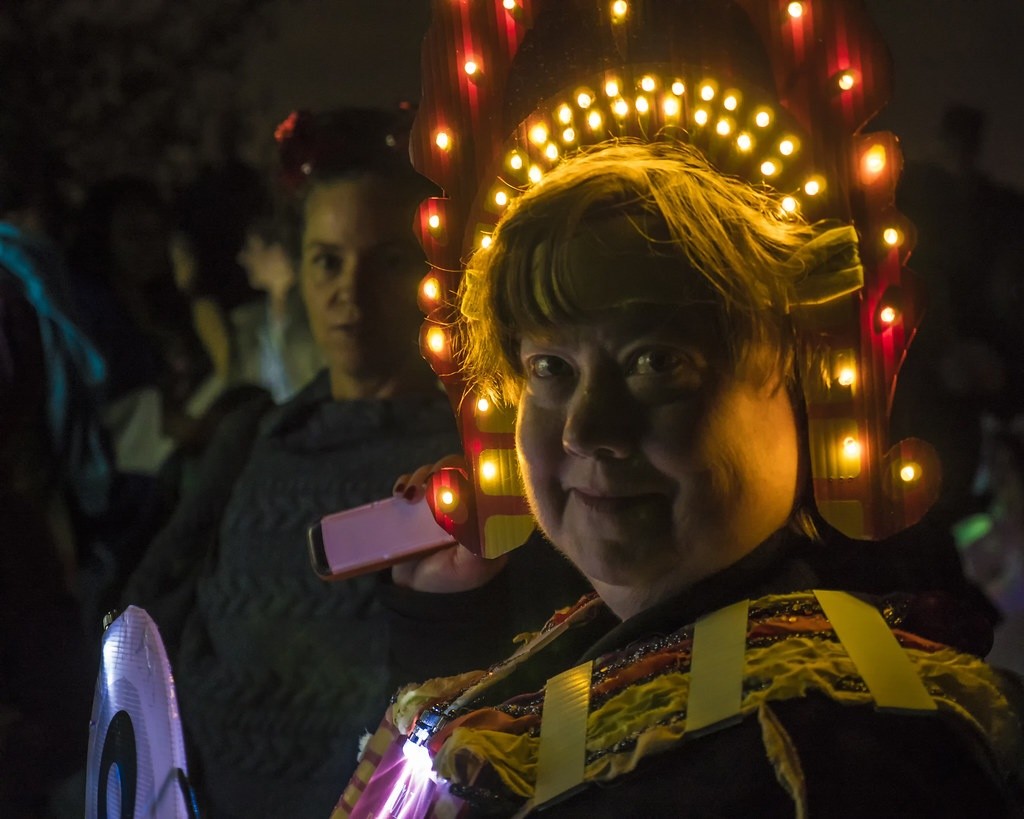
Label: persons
xmin=390 ymin=63 xmax=1024 ymax=819
xmin=0 ymin=105 xmax=464 ymax=819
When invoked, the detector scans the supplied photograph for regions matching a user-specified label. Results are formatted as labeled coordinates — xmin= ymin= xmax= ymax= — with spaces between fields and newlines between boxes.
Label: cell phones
xmin=308 ymin=488 xmax=458 ymax=581
